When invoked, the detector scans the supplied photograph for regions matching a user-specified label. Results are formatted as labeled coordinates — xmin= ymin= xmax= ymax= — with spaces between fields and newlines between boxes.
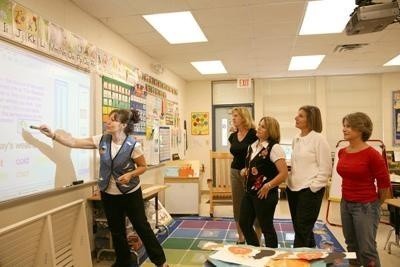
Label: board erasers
xmin=72 ymin=180 xmax=84 ymax=185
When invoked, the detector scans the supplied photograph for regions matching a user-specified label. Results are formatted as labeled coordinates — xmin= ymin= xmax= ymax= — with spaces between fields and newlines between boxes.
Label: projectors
xmin=344 ymin=0 xmax=400 ymax=36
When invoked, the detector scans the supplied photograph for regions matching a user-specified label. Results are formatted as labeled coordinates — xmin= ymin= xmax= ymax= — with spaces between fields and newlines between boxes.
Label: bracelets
xmin=52 ymin=131 xmax=57 ymax=140
xmin=264 ymin=182 xmax=273 ymax=191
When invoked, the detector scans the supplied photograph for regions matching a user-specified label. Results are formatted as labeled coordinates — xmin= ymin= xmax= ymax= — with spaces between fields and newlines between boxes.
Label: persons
xmin=281 ymin=104 xmax=333 ymax=247
xmin=237 ymin=116 xmax=289 ymax=247
xmin=227 ymin=104 xmax=258 ymax=246
xmin=336 ymin=110 xmax=391 ymax=267
xmin=39 ymin=107 xmax=170 ymax=266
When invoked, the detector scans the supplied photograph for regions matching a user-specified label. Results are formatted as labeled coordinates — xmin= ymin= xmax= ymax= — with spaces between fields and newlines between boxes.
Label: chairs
xmin=207 ymin=150 xmax=235 ymax=218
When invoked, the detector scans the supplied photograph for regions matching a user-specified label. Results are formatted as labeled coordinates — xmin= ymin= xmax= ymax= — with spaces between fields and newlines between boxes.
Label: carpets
xmin=112 ymin=213 xmax=347 ymax=267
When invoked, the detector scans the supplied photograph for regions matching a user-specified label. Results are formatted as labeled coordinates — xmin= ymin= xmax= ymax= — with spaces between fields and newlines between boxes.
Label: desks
xmin=204 ymin=242 xmax=330 ymax=267
xmin=383 ymin=196 xmax=400 ymax=257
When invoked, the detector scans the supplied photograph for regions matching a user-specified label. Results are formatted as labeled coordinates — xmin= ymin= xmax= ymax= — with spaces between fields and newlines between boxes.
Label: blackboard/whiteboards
xmin=327 ymin=140 xmax=383 ymax=203
xmin=0 ymin=32 xmax=99 ymax=208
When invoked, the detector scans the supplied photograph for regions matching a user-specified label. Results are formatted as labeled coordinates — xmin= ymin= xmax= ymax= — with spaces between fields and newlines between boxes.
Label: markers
xmin=63 ymin=185 xmax=72 ymax=187
xmin=30 ymin=126 xmax=41 ymax=129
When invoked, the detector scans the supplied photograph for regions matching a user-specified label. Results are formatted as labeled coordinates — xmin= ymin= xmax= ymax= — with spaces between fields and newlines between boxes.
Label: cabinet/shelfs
xmin=386 ymin=149 xmax=400 ymax=176
xmin=85 ymin=182 xmax=168 ymax=266
xmin=163 ymin=169 xmax=205 ymax=217
